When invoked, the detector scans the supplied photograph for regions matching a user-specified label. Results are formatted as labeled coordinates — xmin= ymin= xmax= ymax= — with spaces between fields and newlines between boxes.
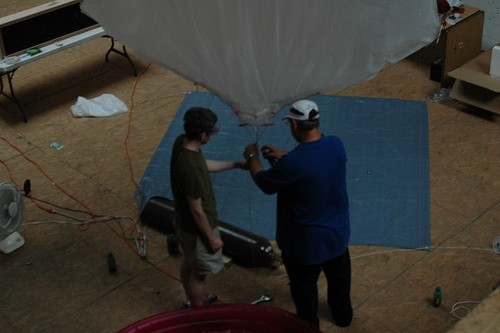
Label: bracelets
xmin=248 ymin=153 xmax=257 ymax=159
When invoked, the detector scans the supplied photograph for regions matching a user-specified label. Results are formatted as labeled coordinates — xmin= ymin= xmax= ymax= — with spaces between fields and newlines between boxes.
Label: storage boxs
xmin=490 ymin=45 xmax=500 ymax=77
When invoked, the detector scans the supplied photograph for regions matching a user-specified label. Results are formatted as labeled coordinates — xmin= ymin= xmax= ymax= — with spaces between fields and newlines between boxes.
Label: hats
xmin=281 ymin=100 xmax=320 ymax=121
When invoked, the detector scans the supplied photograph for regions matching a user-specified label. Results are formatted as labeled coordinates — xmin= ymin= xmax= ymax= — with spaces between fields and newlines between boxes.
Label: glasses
xmin=210 ymin=128 xmax=219 ymax=134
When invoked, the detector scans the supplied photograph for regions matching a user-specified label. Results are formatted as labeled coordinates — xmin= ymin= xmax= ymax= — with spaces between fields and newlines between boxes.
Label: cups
xmin=167 ymin=234 xmax=179 ymax=256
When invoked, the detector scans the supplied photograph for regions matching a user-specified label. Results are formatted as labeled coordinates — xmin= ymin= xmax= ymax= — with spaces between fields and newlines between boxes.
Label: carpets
xmin=135 ymin=90 xmax=431 ymax=251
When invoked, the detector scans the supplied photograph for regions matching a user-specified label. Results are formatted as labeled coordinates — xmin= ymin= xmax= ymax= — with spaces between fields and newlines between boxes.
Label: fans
xmin=0 ymin=182 xmax=26 ymax=254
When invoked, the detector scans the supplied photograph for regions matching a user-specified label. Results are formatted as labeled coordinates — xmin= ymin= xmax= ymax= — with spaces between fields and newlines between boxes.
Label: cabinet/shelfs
xmin=429 ymin=5 xmax=485 ymax=83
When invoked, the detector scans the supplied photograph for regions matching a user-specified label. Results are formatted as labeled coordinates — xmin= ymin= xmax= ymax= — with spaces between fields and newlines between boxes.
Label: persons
xmin=242 ymin=99 xmax=354 ymax=333
xmin=169 ymin=106 xmax=250 ymax=307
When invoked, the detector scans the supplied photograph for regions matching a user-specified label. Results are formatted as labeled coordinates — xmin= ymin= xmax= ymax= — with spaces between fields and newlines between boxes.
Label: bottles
xmin=433 ymin=286 xmax=441 ymax=307
xmin=106 ymin=252 xmax=117 ymax=272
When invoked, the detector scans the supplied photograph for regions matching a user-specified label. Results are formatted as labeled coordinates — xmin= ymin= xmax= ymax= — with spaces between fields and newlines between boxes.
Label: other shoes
xmin=182 ymin=293 xmax=218 ymax=308
